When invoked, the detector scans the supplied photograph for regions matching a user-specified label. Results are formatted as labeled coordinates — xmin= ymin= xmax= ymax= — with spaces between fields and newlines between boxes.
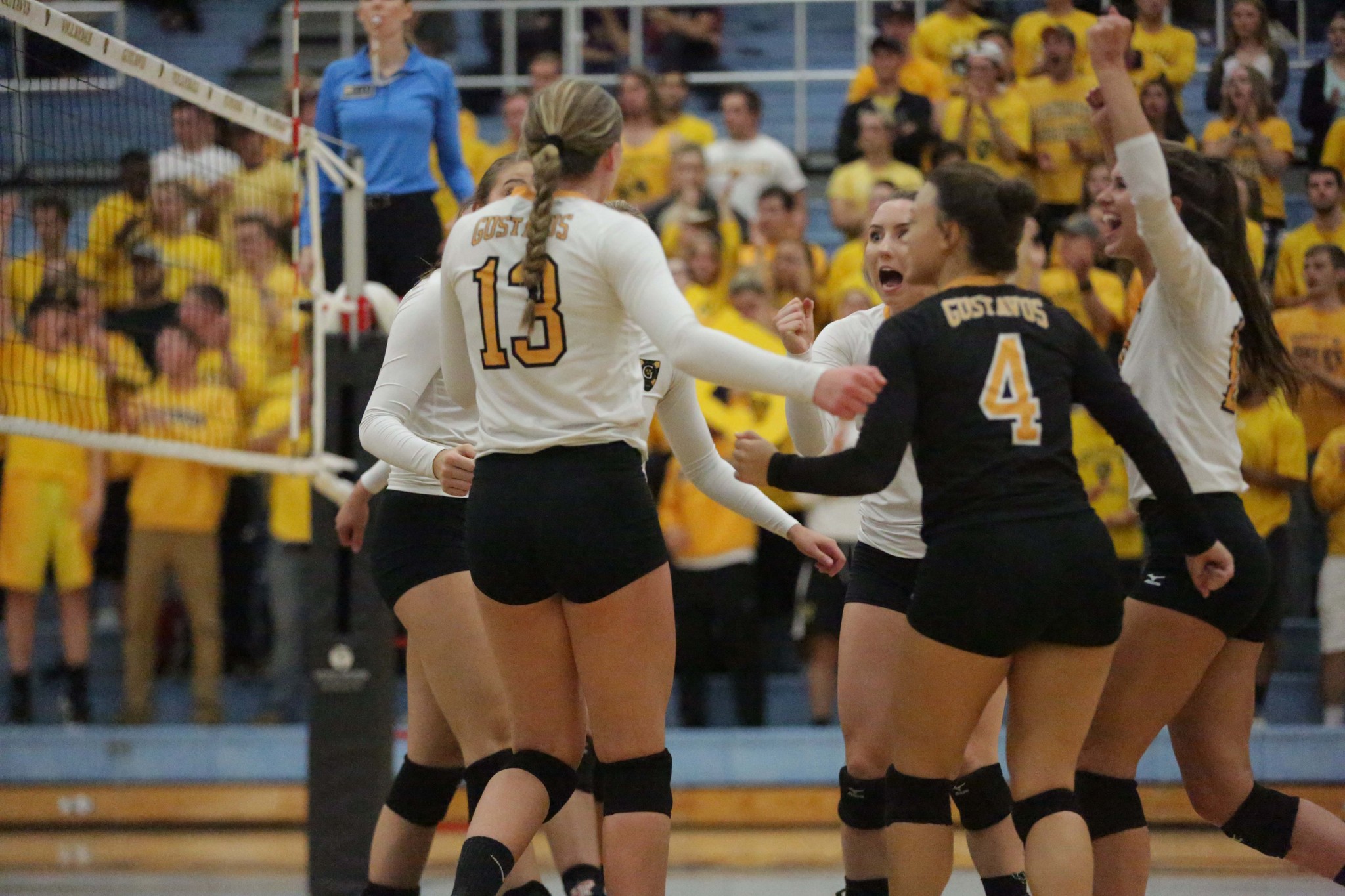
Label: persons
xmin=0 ymin=0 xmax=1345 ymax=896
xmin=106 ymin=321 xmax=241 ymax=726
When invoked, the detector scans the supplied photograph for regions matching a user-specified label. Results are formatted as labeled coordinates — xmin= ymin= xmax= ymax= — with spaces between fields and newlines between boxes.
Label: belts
xmin=364 ymin=192 xmax=423 ymax=211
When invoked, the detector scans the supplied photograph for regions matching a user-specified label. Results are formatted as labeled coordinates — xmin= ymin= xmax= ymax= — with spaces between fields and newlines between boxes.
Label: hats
xmin=873 ymin=1 xmax=916 ymax=27
xmin=129 ymin=239 xmax=161 ymax=263
xmin=869 ymin=35 xmax=905 ymax=56
